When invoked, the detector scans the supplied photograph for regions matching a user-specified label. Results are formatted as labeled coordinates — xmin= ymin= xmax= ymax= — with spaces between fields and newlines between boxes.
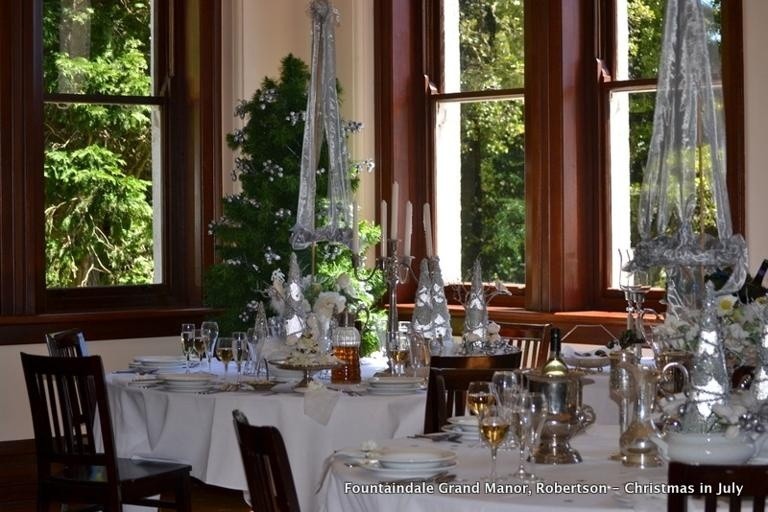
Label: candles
xmin=352 ymin=200 xmax=361 ymax=255
xmin=380 ymin=199 xmax=388 ymax=258
xmin=404 ymin=200 xmax=413 ymax=257
xmin=391 ymin=179 xmax=399 ymax=239
xmin=423 ymin=203 xmax=435 ymax=258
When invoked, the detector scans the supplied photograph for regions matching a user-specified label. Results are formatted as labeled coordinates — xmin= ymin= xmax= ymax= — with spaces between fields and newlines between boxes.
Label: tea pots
xmin=609 ymin=356 xmax=687 ymax=467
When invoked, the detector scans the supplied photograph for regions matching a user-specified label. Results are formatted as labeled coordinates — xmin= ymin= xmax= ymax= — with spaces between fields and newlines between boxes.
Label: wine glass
xmin=216 ymin=337 xmax=235 ymax=379
xmin=247 ymin=329 xmax=262 ymax=375
xmin=466 ymin=381 xmax=495 ymax=449
xmin=608 ymin=352 xmax=639 ymax=462
xmin=388 ymin=341 xmax=407 ymax=376
xmin=181 ymin=323 xmax=195 ymax=374
xmin=478 ymin=410 xmax=510 ymax=484
xmin=201 ymin=321 xmax=218 ymax=372
xmin=492 ymin=370 xmax=516 ymax=449
xmin=193 ymin=328 xmax=204 ymax=373
xmin=512 ymin=394 xmax=548 ymax=481
xmin=231 ymin=332 xmax=246 ymax=375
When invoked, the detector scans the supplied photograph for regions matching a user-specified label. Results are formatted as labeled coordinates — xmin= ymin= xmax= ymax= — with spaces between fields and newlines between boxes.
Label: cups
xmin=527 ymin=372 xmax=584 ymax=465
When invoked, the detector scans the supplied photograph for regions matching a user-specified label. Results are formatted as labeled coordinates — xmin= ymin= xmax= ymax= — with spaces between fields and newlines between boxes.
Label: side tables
xmin=311 ymin=354 xmax=766 ymax=511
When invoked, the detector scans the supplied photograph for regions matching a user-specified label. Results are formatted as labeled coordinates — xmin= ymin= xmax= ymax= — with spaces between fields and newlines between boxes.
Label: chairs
xmin=490 ymin=322 xmax=552 ymax=387
xmin=19 ymin=351 xmax=192 ymax=512
xmin=666 ymin=459 xmax=767 ymax=511
xmin=423 ymin=350 xmax=523 ymax=436
xmin=231 ymin=408 xmax=301 ymax=512
xmin=43 ymin=327 xmax=140 ymax=472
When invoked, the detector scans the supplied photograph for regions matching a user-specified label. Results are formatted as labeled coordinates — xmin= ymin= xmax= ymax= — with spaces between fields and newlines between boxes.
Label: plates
xmin=375 ymin=447 xmax=456 ymax=466
xmin=155 ymin=382 xmax=214 ymax=393
xmin=360 ymin=461 xmax=459 ymax=480
xmin=128 ymin=362 xmax=181 ymax=372
xmin=564 ymin=357 xmax=610 ymax=368
xmin=156 ymin=374 xmax=216 ymax=384
xmin=439 ymin=424 xmax=482 ymax=441
xmin=446 ymin=416 xmax=483 ymax=433
xmin=132 ymin=356 xmax=182 ymax=365
xmin=368 ymin=377 xmax=425 ymax=389
xmin=366 ymin=387 xmax=425 ymax=395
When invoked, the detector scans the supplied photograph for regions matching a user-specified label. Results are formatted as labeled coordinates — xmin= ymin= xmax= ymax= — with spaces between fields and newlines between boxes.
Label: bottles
xmin=331 ymin=311 xmax=362 ymax=384
xmin=541 ymin=328 xmax=568 ymax=377
